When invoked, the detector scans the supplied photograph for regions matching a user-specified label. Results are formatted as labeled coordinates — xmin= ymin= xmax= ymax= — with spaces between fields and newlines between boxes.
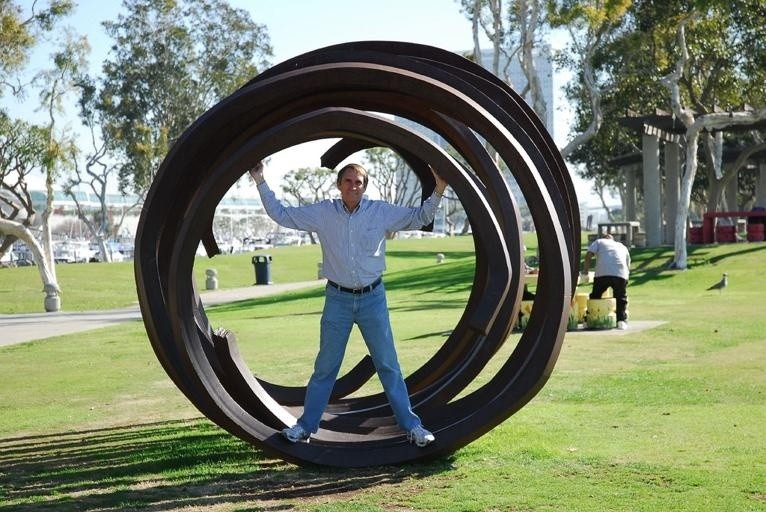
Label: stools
xmin=587 ymin=233 xmax=646 ymax=248
xmin=519 ymin=293 xmax=618 ymax=331
xmin=690 ymin=225 xmax=763 ymax=244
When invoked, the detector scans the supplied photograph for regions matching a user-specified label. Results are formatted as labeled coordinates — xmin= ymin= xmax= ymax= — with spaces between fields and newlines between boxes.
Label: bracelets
xmin=434 ymin=191 xmax=442 ymax=199
xmin=582 ymin=270 xmax=588 ymax=274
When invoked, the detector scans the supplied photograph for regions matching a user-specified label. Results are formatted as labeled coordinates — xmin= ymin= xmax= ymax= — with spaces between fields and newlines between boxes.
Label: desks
xmin=597 ymin=223 xmax=640 ymax=249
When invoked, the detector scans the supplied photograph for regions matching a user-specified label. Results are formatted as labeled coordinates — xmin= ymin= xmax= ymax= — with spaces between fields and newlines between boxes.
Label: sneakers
xmin=583 ymin=321 xmax=588 ymax=329
xmin=281 ymin=422 xmax=310 ymax=444
xmin=618 ymin=321 xmax=627 ymax=330
xmin=407 ymin=424 xmax=436 ymax=447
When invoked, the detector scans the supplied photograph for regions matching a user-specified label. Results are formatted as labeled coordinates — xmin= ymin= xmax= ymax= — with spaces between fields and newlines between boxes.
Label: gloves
xmin=582 ymin=273 xmax=590 ymax=284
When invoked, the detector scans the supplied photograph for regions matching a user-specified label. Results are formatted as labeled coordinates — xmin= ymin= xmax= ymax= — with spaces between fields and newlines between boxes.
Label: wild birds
xmin=706 ymin=272 xmax=728 ymax=293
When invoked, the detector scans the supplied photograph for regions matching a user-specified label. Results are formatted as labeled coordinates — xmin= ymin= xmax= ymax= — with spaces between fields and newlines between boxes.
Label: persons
xmin=580 ymin=233 xmax=630 ymax=330
xmin=249 ymin=161 xmax=448 ymax=446
xmin=513 ymin=242 xmax=539 ymax=331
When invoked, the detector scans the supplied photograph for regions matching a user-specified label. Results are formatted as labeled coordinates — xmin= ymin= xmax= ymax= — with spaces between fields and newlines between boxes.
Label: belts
xmin=328 ymin=276 xmax=381 ymax=293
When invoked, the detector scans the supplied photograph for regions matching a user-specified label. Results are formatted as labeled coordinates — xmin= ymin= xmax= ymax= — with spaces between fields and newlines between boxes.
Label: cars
xmin=0 ymin=235 xmax=134 ymax=265
xmin=196 ymin=227 xmax=320 ymax=255
xmin=393 ymin=229 xmax=447 ymax=239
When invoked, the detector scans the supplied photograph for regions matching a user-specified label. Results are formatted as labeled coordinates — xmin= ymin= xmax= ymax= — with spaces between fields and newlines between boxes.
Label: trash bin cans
xmin=635 ymin=233 xmax=645 ymax=248
xmin=252 ymin=255 xmax=272 ymax=284
